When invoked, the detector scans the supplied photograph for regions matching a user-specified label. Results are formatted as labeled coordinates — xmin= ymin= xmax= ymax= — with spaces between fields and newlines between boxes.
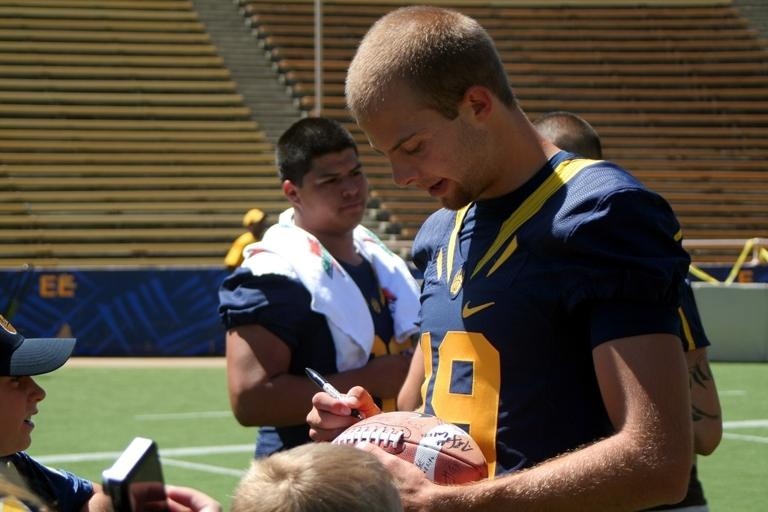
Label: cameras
xmin=101 ymin=439 xmax=168 ymax=511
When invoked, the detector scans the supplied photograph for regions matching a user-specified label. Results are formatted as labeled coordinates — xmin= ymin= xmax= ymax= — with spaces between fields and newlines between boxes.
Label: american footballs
xmin=332 ymin=412 xmax=488 ymax=485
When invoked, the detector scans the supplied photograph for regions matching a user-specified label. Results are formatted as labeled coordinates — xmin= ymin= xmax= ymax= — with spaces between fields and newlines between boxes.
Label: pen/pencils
xmin=304 ymin=367 xmax=364 ymax=421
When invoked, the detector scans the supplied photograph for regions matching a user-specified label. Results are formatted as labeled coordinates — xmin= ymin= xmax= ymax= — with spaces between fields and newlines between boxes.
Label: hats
xmin=242 ymin=208 xmax=266 ymax=228
xmin=0 ymin=314 xmax=77 ymax=377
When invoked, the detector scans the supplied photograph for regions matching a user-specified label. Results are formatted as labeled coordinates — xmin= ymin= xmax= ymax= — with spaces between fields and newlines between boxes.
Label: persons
xmin=227 ymin=435 xmax=400 ymax=509
xmin=343 ymin=3 xmax=692 ymax=509
xmin=528 ymin=106 xmax=724 ymax=510
xmin=217 ymin=206 xmax=270 ymax=274
xmin=1 ymin=314 xmax=226 ymax=512
xmin=218 ymin=113 xmax=425 ymax=464
